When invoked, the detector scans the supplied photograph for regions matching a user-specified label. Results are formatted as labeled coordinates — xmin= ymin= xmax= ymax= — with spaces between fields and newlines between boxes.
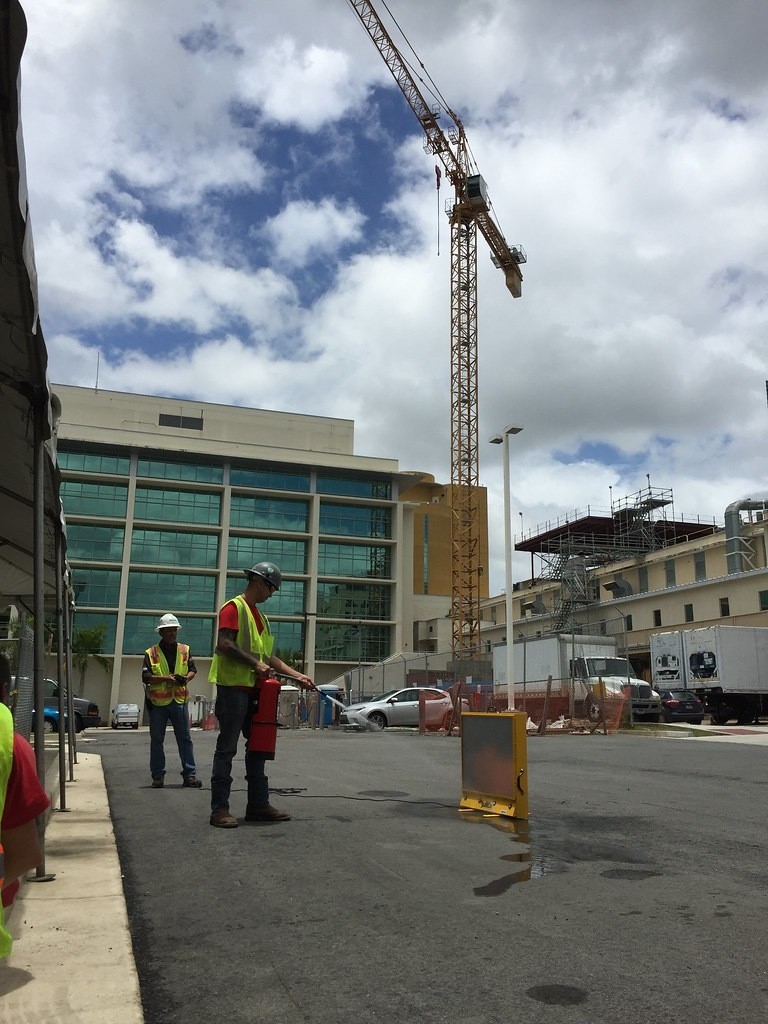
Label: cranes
xmin=350 ymin=0 xmax=526 ymax=724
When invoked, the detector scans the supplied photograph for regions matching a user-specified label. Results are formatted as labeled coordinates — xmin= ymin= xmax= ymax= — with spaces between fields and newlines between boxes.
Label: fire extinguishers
xmin=248 ymin=667 xmax=328 ymax=761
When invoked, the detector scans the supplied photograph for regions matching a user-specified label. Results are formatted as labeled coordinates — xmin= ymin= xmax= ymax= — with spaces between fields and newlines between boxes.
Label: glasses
xmin=263 ymin=579 xmax=276 ymax=593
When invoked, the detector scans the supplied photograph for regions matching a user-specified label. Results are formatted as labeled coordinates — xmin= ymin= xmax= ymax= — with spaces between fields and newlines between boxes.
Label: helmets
xmin=243 ymin=562 xmax=282 ymax=590
xmin=155 ymin=613 xmax=183 ymax=633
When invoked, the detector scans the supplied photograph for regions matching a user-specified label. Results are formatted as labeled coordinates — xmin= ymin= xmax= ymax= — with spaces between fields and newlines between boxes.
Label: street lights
xmin=487 ymin=424 xmax=524 ymax=711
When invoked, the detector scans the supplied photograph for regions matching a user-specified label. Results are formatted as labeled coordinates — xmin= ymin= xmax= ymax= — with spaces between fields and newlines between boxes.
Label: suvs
xmin=111 ymin=704 xmax=141 ymax=730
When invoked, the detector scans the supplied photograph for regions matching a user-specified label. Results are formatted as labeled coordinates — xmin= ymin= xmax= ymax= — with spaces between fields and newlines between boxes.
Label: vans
xmin=8 ymin=677 xmax=102 ymax=734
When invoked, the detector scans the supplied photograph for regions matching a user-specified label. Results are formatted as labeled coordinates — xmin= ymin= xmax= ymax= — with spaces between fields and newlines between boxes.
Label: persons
xmin=142 ymin=613 xmax=202 ymax=788
xmin=207 ymin=562 xmax=316 ymax=828
xmin=0 ymin=652 xmax=50 ymax=957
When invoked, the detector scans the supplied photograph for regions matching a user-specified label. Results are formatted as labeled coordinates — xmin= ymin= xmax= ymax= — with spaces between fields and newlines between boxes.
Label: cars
xmin=31 ymin=705 xmax=70 ymax=733
xmin=338 ymin=686 xmax=471 ymax=734
xmin=657 ymin=690 xmax=705 ymax=725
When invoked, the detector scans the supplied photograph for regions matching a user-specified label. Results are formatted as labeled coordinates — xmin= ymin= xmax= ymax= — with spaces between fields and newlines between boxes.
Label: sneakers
xmin=183 ymin=778 xmax=202 ymax=788
xmin=210 ymin=810 xmax=238 ymax=828
xmin=152 ymin=779 xmax=164 ymax=788
xmin=245 ymin=804 xmax=291 ymax=822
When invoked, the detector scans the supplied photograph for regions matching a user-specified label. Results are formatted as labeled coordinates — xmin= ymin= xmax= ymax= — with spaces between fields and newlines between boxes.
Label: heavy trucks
xmin=491 ymin=633 xmax=662 ymax=724
